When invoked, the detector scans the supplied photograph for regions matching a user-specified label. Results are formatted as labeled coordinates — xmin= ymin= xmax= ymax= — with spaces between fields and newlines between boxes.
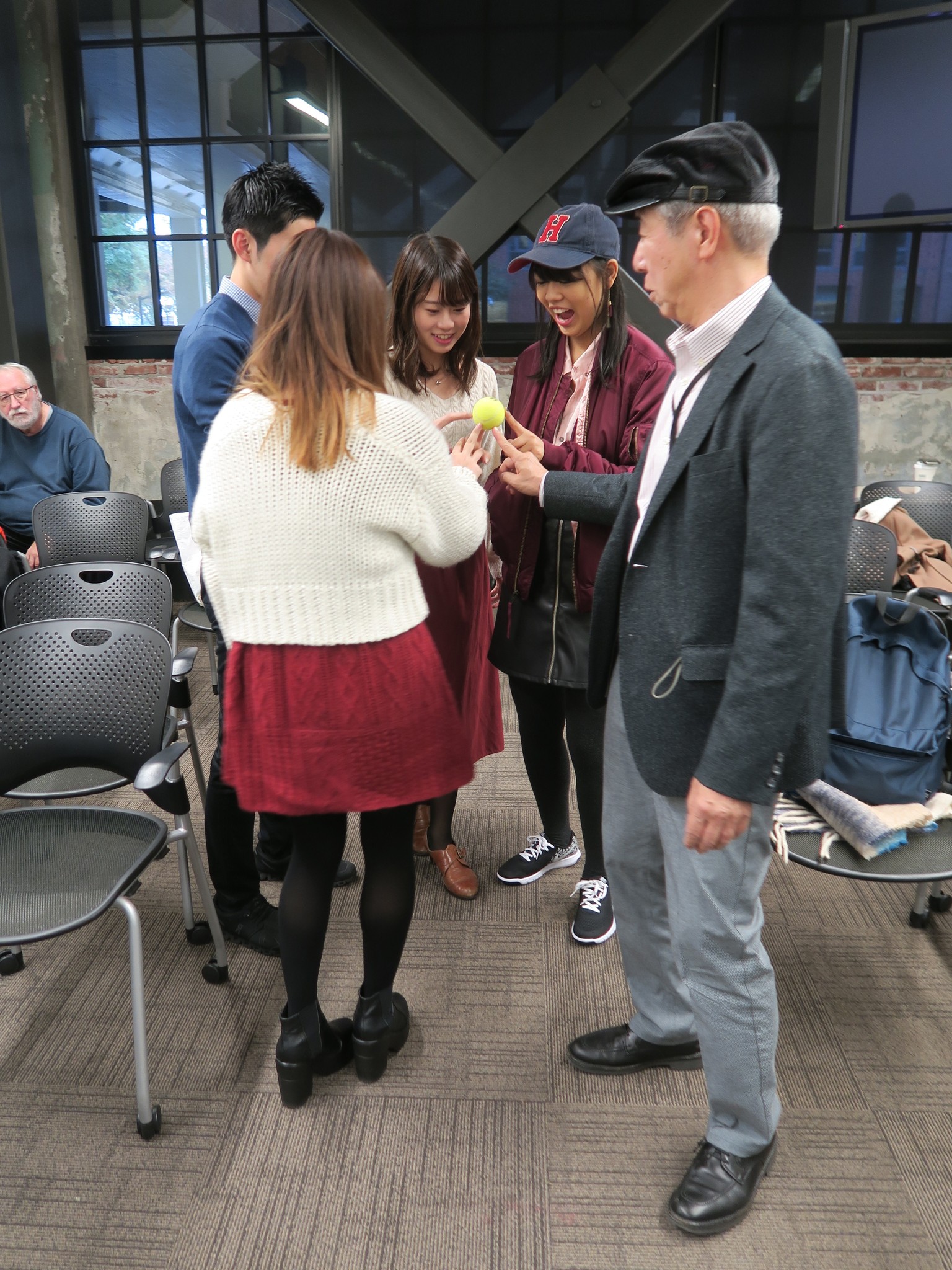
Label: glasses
xmin=0 ymin=386 xmax=34 ymax=406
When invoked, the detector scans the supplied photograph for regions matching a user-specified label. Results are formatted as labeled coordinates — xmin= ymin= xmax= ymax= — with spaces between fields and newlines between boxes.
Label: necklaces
xmin=431 ymin=372 xmax=445 ymax=385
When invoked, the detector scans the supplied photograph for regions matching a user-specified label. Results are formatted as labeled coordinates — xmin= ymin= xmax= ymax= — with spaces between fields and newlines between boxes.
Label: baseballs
xmin=472 ymin=397 xmax=506 ymax=430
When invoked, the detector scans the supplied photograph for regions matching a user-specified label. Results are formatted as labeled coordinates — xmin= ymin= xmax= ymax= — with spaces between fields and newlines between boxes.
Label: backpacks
xmin=820 ymin=595 xmax=952 ymax=806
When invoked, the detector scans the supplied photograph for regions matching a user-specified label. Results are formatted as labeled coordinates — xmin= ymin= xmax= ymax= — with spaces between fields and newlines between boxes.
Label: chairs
xmin=760 ymin=473 xmax=952 ymax=934
xmin=0 ymin=455 xmax=234 ymax=1146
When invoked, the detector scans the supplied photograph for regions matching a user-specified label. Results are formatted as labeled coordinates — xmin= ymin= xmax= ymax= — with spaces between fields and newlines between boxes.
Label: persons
xmin=488 ymin=120 xmax=860 ymax=1238
xmin=494 ymin=204 xmax=676 ymax=950
xmin=0 ymin=359 xmax=110 ymax=570
xmin=172 ymin=163 xmax=507 ymax=1114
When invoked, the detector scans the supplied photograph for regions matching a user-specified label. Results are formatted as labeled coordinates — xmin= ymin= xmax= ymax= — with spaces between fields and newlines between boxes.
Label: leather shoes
xmin=567 ymin=1024 xmax=705 ymax=1070
xmin=669 ymin=1133 xmax=778 ymax=1234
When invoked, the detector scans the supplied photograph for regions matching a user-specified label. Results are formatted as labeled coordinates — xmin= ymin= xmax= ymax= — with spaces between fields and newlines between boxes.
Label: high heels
xmin=352 ymin=989 xmax=410 ymax=1083
xmin=275 ymin=999 xmax=353 ymax=1109
xmin=423 ymin=827 xmax=480 ymax=901
xmin=413 ymin=804 xmax=430 ymax=856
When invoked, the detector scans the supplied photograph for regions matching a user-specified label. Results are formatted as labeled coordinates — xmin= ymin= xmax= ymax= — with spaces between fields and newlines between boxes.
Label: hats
xmin=508 ymin=203 xmax=620 ymax=274
xmin=605 ymin=120 xmax=780 ymax=216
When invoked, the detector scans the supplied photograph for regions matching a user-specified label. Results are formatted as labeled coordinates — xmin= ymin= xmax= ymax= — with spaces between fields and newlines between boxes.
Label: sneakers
xmin=214 ymin=896 xmax=282 ymax=957
xmin=569 ymin=875 xmax=616 ymax=945
xmin=255 ymin=842 xmax=357 ymax=887
xmin=497 ymin=831 xmax=581 ymax=885
